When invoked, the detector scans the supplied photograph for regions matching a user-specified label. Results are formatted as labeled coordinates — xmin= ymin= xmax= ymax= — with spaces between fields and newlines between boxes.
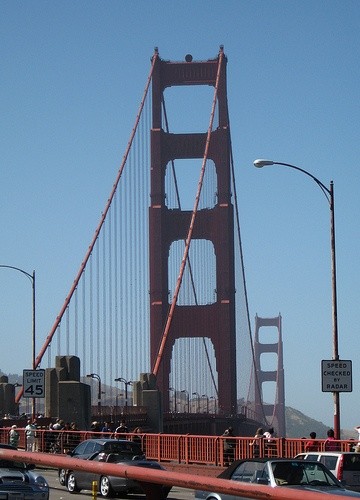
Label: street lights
xmin=114 ymin=377 xmax=127 ymax=406
xmin=86 ymin=373 xmax=102 ymax=406
xmin=192 ymin=392 xmax=200 ymax=413
xmin=169 ymin=387 xmax=176 ymax=413
xmin=252 ymin=158 xmax=341 ymax=441
xmin=181 ymin=390 xmax=189 ymax=413
xmin=202 ymin=394 xmax=208 ymax=414
xmin=210 ymin=396 xmax=216 ymax=414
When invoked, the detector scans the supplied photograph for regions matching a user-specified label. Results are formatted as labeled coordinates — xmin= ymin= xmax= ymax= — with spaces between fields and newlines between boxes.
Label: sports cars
xmin=64 ymin=449 xmax=174 ymax=500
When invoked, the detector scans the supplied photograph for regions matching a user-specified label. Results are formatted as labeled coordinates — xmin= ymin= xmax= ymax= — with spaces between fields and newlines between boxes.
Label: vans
xmin=290 ymin=451 xmax=360 ymax=492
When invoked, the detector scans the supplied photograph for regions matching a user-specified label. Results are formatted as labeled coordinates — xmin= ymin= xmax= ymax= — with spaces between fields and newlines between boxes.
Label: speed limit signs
xmin=22 ymin=369 xmax=45 ymax=398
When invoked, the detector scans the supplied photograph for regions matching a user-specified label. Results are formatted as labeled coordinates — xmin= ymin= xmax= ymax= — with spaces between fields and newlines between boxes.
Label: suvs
xmin=57 ymin=438 xmax=148 ymax=487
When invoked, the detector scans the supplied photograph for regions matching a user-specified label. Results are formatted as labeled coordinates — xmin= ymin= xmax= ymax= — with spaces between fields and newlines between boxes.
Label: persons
xmin=102 ymin=423 xmax=112 ymax=439
xmin=25 ymin=419 xmax=39 ymax=452
xmin=221 ymin=427 xmax=236 ymax=467
xmin=130 ymin=427 xmax=143 ymax=451
xmin=353 ymin=426 xmax=360 ymax=452
xmin=304 ymin=432 xmax=321 ymax=452
xmin=322 ymin=429 xmax=341 ymax=452
xmin=7 ymin=425 xmax=20 ymax=446
xmin=248 ymin=428 xmax=263 ymax=458
xmin=48 ymin=418 xmax=81 ymax=453
xmin=263 ymin=428 xmax=275 ymax=442
xmin=115 ymin=420 xmax=129 ymax=450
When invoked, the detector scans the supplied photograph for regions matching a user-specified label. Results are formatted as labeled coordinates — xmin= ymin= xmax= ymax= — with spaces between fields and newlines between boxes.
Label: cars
xmin=0 ymin=443 xmax=50 ymax=500
xmin=194 ymin=457 xmax=360 ymax=500
